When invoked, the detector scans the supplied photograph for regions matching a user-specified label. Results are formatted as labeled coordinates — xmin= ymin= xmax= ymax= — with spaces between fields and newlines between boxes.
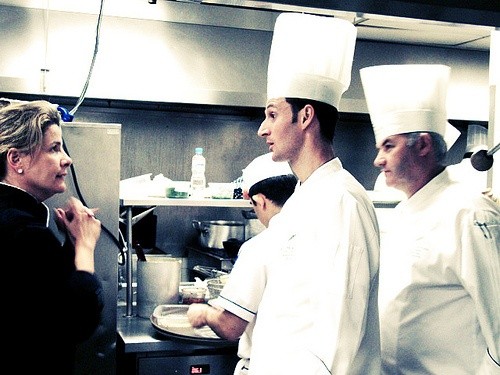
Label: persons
xmin=250 ymin=63 xmax=499 ymax=375
xmin=0 ymin=100 xmax=104 ymax=375
xmin=186 ymin=152 xmax=298 ymax=375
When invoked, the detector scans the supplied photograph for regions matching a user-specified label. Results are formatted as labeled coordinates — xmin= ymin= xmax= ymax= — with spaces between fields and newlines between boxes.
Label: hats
xmin=359 ymin=64 xmax=451 ymax=148
xmin=245 ymin=154 xmax=294 ymax=194
xmin=266 ymin=13 xmax=357 ymax=112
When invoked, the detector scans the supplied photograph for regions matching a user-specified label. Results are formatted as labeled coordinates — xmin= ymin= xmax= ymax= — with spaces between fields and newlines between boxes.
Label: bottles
xmin=190 ymin=148 xmax=207 ymax=191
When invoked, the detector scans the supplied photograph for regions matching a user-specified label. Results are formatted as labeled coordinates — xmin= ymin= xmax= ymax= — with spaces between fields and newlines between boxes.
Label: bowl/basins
xmin=180 ymin=278 xmax=230 ymax=306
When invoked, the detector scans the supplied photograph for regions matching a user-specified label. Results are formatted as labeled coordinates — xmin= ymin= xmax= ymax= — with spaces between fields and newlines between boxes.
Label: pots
xmin=242 ymin=209 xmax=266 ymax=240
xmin=191 ymin=219 xmax=245 ymax=251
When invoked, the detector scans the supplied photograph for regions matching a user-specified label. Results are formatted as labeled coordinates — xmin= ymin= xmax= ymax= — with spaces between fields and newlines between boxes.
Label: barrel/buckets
xmin=137 ymin=258 xmax=181 ymax=318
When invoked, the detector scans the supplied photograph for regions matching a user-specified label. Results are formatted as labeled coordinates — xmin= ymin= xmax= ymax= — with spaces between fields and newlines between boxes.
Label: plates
xmin=149 ymin=303 xmax=241 ymax=343
